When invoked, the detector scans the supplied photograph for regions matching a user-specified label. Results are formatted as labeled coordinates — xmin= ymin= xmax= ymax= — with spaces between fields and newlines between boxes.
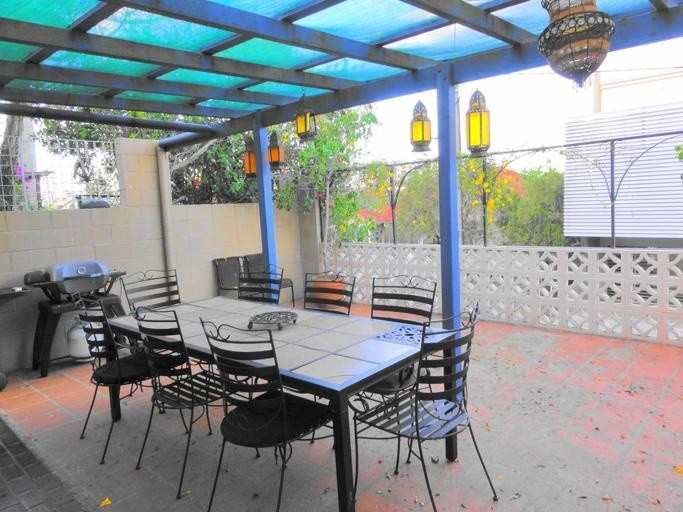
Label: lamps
xmin=410 ymin=101 xmax=430 ymax=154
xmin=268 ymin=133 xmax=285 ymax=170
xmin=539 ymin=0 xmax=614 ymax=87
xmin=295 ymin=108 xmax=317 ymax=143
xmin=243 ymin=139 xmax=258 ymax=179
xmin=466 ymin=90 xmax=488 ymax=159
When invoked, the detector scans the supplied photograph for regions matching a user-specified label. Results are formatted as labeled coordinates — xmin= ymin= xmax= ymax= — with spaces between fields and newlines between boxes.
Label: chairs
xmin=197 ymin=317 xmax=333 ymax=511
xmin=135 ymin=307 xmax=259 ymax=500
xmin=328 ymin=275 xmax=436 ymax=475
xmin=70 ymin=301 xmax=172 ymax=465
xmin=278 ymin=273 xmax=357 ymax=444
xmin=353 ymin=303 xmax=498 ymax=512
xmin=222 ymin=264 xmax=283 ymax=402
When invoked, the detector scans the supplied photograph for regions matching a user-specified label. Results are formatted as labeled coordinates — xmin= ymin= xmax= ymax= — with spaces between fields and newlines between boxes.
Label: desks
xmin=110 ymin=296 xmax=457 ymax=512
